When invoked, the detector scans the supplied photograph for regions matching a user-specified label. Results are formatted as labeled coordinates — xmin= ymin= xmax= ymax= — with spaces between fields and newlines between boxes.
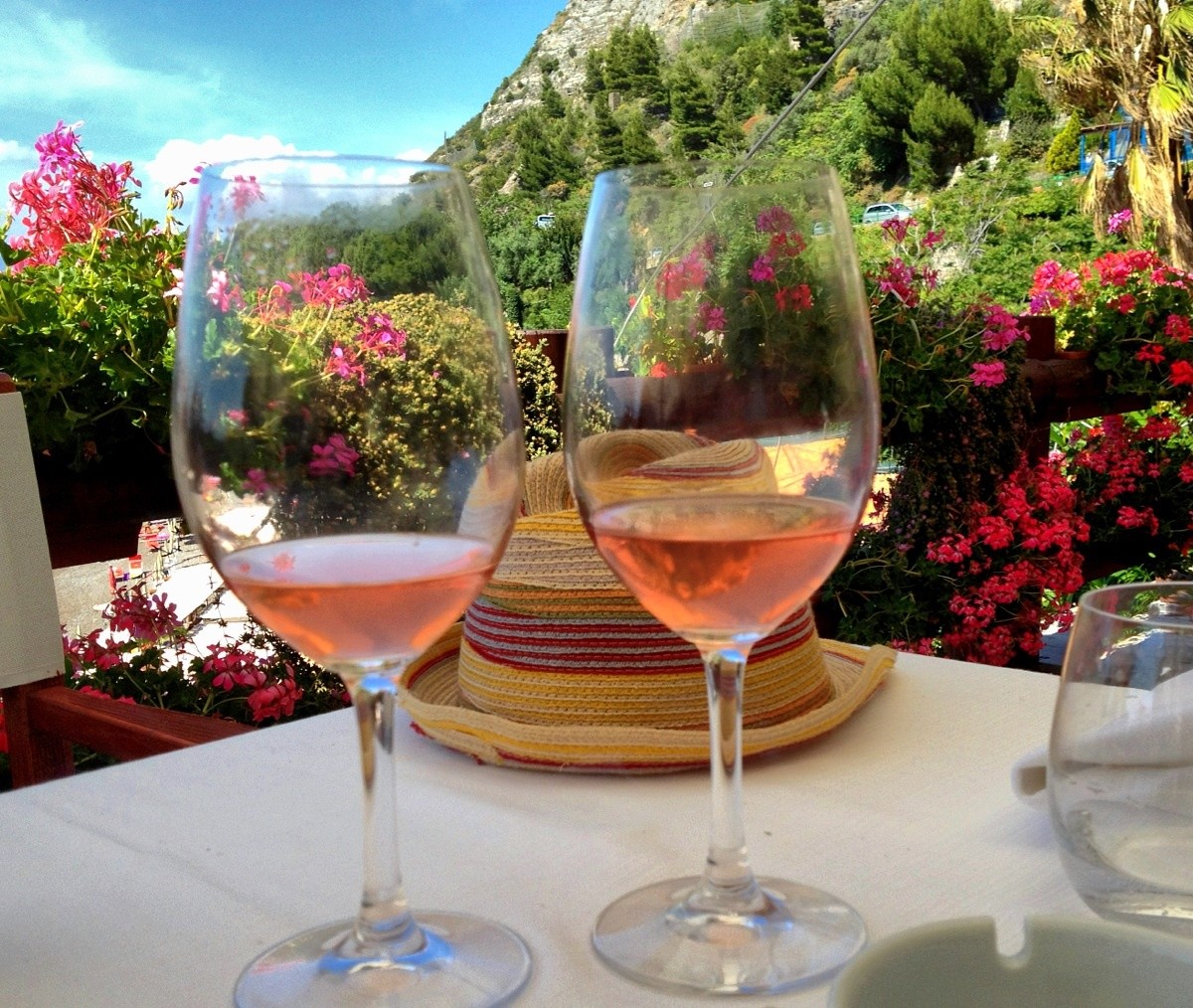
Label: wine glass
xmin=169 ymin=157 xmax=525 ymax=1006
xmin=562 ymin=159 xmax=887 ymax=1004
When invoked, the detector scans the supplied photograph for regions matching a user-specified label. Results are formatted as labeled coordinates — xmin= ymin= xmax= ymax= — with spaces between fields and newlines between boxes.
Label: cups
xmin=1042 ymin=574 xmax=1192 ymax=924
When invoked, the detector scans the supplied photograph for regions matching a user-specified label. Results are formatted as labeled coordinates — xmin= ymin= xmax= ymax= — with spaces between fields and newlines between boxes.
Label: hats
xmin=396 ymin=426 xmax=900 ymax=775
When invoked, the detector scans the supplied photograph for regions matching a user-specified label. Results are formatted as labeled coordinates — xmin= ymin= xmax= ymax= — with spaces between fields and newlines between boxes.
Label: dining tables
xmin=0 ymin=643 xmax=1191 ymax=1008
